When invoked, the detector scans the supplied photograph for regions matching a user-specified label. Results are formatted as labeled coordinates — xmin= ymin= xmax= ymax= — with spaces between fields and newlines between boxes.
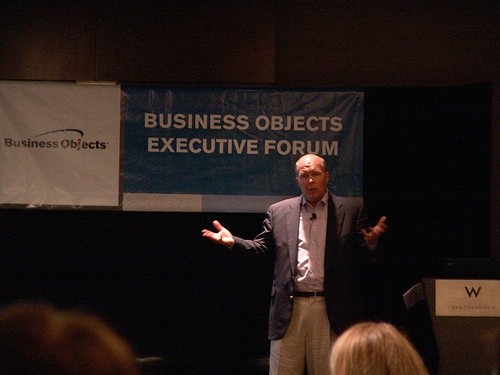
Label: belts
xmin=294 ymin=291 xmax=324 ymax=297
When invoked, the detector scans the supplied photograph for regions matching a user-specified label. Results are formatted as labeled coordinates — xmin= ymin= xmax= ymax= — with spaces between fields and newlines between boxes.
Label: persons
xmin=330 ymin=322 xmax=428 ymax=375
xmin=202 ymin=154 xmax=386 ymax=375
